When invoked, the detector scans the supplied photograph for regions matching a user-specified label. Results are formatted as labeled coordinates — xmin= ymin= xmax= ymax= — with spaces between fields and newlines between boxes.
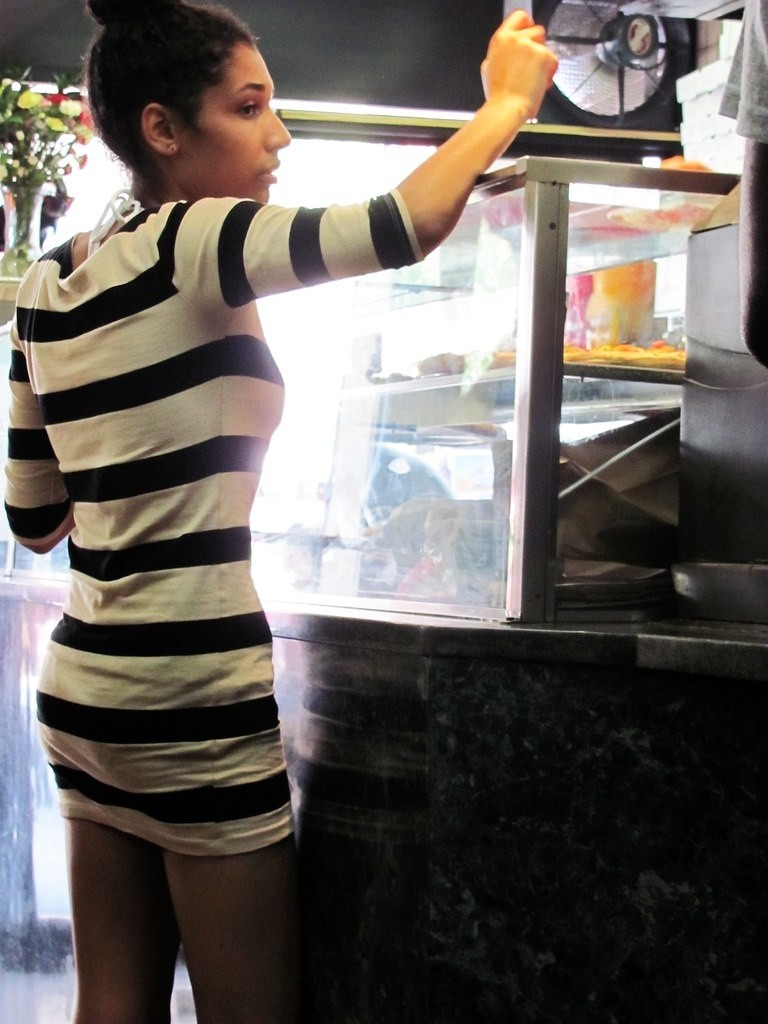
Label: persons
xmin=3 ymin=1 xmax=559 ymax=1024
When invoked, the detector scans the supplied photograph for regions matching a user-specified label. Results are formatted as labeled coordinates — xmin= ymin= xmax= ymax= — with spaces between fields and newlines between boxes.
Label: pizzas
xmin=418 ymin=342 xmax=688 ymax=370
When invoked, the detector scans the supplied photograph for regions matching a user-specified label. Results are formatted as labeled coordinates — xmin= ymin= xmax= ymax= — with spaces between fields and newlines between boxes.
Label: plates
xmin=551 ymin=556 xmax=665 ymax=601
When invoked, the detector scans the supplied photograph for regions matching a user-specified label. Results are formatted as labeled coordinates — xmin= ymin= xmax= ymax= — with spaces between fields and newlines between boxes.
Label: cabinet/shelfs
xmin=319 ymin=155 xmax=742 ymax=621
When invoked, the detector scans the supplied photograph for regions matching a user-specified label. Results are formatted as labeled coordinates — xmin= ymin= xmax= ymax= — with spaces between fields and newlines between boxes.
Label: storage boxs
xmin=676 ymin=55 xmax=744 ymax=171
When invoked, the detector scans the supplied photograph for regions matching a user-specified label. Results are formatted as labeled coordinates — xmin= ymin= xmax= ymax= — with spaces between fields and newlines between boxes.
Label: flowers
xmin=0 ymin=63 xmax=95 ymax=182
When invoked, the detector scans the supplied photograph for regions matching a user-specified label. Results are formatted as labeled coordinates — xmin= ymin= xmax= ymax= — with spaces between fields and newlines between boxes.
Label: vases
xmin=1 ymin=173 xmax=50 ymax=298
